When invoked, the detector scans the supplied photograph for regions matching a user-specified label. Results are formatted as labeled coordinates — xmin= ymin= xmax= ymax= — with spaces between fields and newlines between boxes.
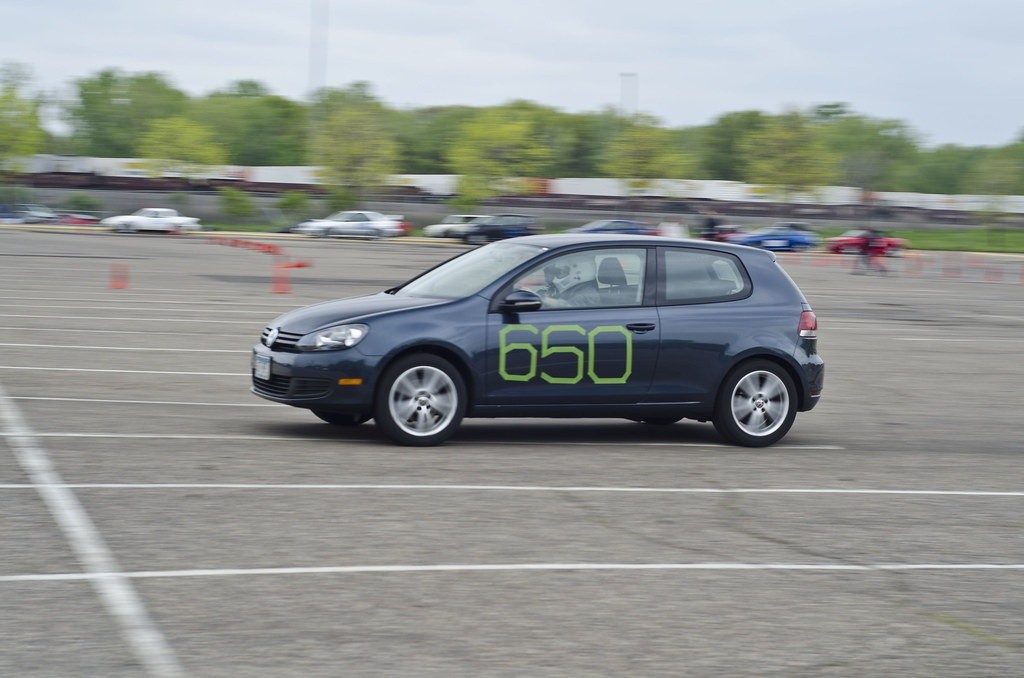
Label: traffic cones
xmin=271 ymin=273 xmax=290 ymax=297
xmin=275 ymin=256 xmax=310 ymax=274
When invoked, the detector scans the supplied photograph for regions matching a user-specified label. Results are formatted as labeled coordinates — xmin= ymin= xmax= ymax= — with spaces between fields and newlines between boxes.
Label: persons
xmin=543 ymin=250 xmax=601 ymax=310
xmin=537 ymin=264 xmax=558 ymax=298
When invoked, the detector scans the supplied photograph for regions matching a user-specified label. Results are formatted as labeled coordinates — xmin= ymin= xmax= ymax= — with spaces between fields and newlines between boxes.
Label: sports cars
xmin=249 ymin=232 xmax=830 ymax=448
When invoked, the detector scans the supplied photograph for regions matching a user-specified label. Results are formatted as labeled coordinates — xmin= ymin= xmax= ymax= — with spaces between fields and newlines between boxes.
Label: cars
xmin=102 ymin=209 xmax=201 ymax=237
xmin=421 ymin=212 xmax=488 ymax=239
xmin=570 ymin=216 xmax=657 ymax=234
xmin=822 ymin=226 xmax=917 ymax=254
xmin=291 ymin=209 xmax=416 ymax=243
xmin=714 ymin=231 xmax=822 ymax=252
xmin=443 ymin=212 xmax=550 ymax=249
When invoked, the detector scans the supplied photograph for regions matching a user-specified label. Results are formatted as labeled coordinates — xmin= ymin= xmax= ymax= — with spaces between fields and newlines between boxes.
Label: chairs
xmin=597 ymin=257 xmax=628 ymax=304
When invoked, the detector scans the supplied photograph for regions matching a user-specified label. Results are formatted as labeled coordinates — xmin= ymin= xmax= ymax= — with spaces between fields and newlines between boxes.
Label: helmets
xmin=551 ymin=253 xmax=597 ymax=294
